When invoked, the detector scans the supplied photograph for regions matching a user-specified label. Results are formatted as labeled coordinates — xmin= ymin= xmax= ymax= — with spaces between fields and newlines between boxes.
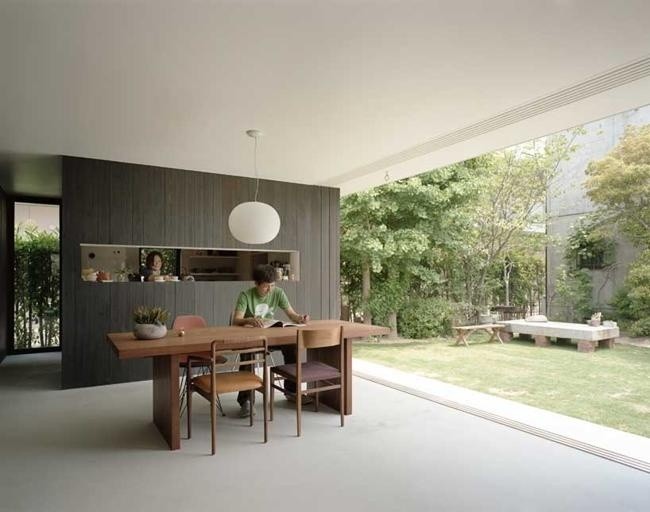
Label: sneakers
xmin=285 ymin=393 xmax=314 ymax=405
xmin=238 ymin=399 xmax=257 ymax=418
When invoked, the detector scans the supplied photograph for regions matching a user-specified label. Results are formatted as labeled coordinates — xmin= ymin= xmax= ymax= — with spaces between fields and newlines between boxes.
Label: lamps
xmin=228 ymin=129 xmax=281 ymax=244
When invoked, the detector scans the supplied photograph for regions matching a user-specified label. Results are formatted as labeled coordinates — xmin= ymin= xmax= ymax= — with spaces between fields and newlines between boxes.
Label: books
xmin=99 ymin=279 xmax=114 ymax=283
xmin=243 ymin=318 xmax=307 ymax=329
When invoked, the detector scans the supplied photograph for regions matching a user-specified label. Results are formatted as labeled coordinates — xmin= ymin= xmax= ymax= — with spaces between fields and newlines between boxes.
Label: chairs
xmin=170 ymin=310 xmax=344 ymax=455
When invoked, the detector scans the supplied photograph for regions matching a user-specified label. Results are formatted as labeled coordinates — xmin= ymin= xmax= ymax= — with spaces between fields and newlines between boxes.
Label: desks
xmin=105 ymin=319 xmax=390 ymax=451
xmin=496 ymin=319 xmax=620 ymax=352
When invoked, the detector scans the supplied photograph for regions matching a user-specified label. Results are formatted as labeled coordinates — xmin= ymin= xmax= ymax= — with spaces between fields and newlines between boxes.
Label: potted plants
xmin=591 ymin=312 xmax=601 ymax=327
xmin=132 ymin=305 xmax=170 ymax=340
xmin=83 ymin=267 xmax=133 ymax=282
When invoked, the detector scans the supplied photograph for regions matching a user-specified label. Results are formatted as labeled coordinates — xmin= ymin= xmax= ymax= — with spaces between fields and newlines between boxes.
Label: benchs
xmin=450 ymin=324 xmax=506 ymax=348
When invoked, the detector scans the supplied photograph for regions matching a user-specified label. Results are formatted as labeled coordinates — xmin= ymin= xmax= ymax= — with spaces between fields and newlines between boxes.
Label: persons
xmin=140 ymin=251 xmax=173 ymax=282
xmin=232 ymin=263 xmax=314 ymax=419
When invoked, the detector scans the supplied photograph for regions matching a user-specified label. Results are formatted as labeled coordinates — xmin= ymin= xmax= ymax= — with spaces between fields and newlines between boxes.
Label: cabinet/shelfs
xmin=189 ymin=255 xmax=240 ymax=276
